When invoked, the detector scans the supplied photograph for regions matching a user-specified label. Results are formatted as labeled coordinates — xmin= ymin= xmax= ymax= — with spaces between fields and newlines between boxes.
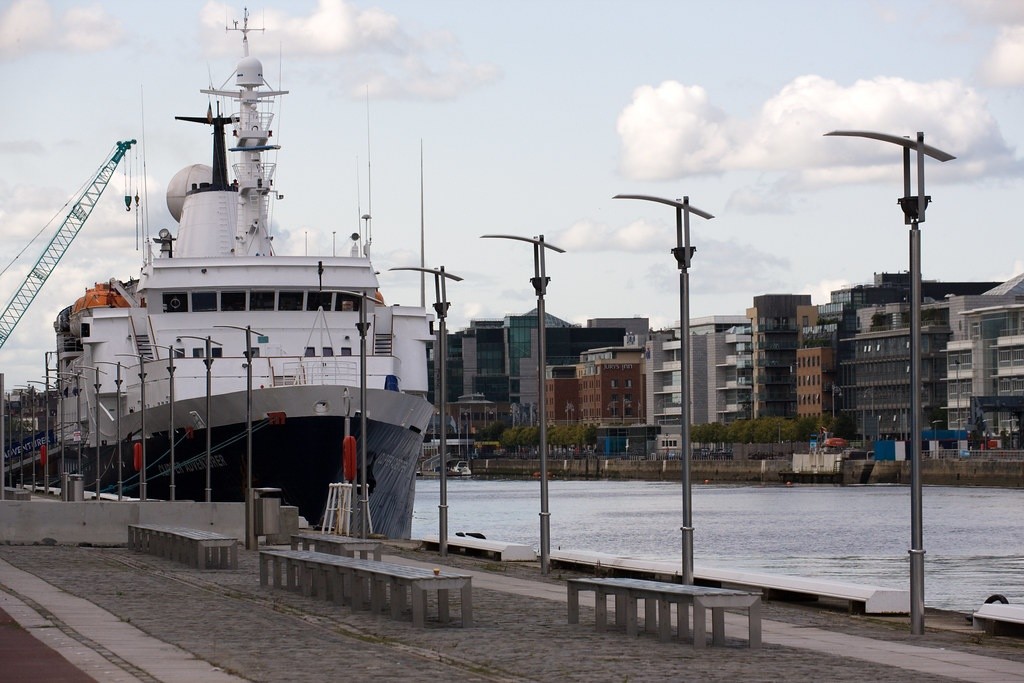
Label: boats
xmin=1 ymin=6 xmax=437 ymax=539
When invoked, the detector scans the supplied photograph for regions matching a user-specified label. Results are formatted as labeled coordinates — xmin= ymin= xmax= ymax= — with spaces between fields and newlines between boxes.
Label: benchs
xmin=290 ymin=533 xmax=383 ymax=562
xmin=260 ymin=551 xmax=474 ymax=629
xmin=128 ymin=524 xmax=238 ymax=570
xmin=566 ymin=579 xmax=764 ymax=649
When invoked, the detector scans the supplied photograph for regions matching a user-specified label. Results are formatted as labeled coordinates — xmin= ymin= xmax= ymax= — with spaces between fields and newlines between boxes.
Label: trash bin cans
xmin=68 ymin=473 xmax=85 ymax=501
xmin=252 ymin=487 xmax=283 ymax=535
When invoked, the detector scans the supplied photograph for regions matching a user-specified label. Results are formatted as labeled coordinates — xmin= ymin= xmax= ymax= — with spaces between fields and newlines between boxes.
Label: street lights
xmin=386 ymin=265 xmax=463 ymax=556
xmin=479 ymin=232 xmax=569 ymax=575
xmin=824 ymin=128 xmax=959 ymax=637
xmin=612 ymin=193 xmax=715 ymax=585
